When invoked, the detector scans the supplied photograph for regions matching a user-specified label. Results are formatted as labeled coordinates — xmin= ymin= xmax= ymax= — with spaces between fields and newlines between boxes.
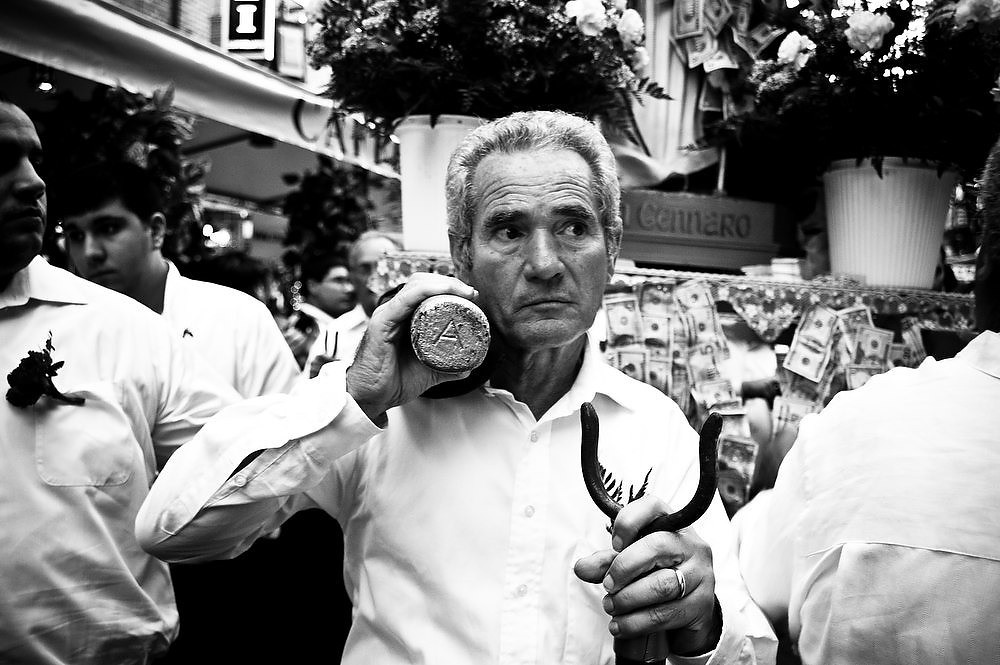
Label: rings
xmin=669 ymin=566 xmax=687 ymax=599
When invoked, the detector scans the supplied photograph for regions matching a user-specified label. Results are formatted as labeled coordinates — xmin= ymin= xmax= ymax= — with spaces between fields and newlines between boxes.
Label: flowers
xmin=677 ymin=0 xmax=1000 ymax=200
xmin=298 ymin=0 xmax=677 ymax=158
xmin=5 ymin=325 xmax=90 ymax=408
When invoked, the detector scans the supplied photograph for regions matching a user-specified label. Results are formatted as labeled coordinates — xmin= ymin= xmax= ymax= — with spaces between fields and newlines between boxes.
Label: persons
xmin=0 ymin=95 xmax=245 ymax=664
xmin=721 ymin=193 xmax=999 ymax=665
xmin=132 ymin=105 xmax=734 ymax=665
xmin=63 ymin=143 xmax=295 ymax=429
xmin=191 ymin=222 xmax=408 ymax=382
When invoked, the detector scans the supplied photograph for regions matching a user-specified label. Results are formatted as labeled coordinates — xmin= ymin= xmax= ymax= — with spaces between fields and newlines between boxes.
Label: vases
xmin=389 ymin=110 xmax=498 ymax=257
xmin=820 ymin=154 xmax=957 ymax=294
